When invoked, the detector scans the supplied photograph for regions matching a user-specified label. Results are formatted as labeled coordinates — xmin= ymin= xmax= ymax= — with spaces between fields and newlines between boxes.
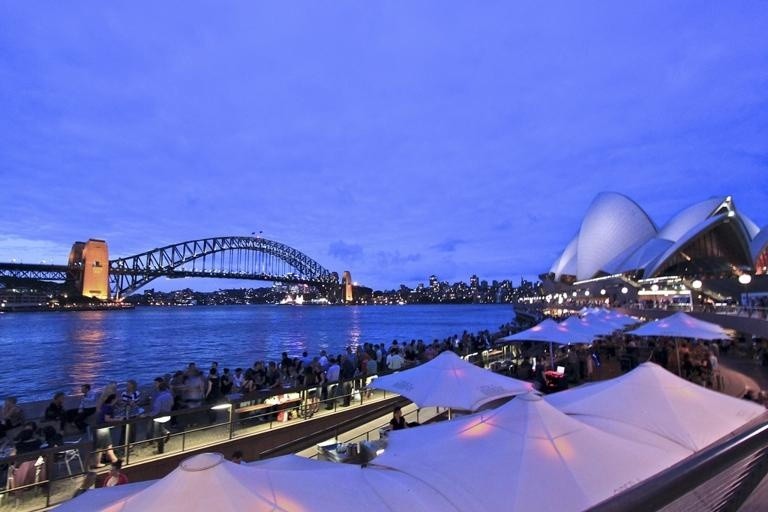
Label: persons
xmin=493 ymin=318 xmax=766 ymax=406
xmin=563 ymin=297 xmax=766 ymax=317
xmin=0 ymin=329 xmax=493 ymax=487
